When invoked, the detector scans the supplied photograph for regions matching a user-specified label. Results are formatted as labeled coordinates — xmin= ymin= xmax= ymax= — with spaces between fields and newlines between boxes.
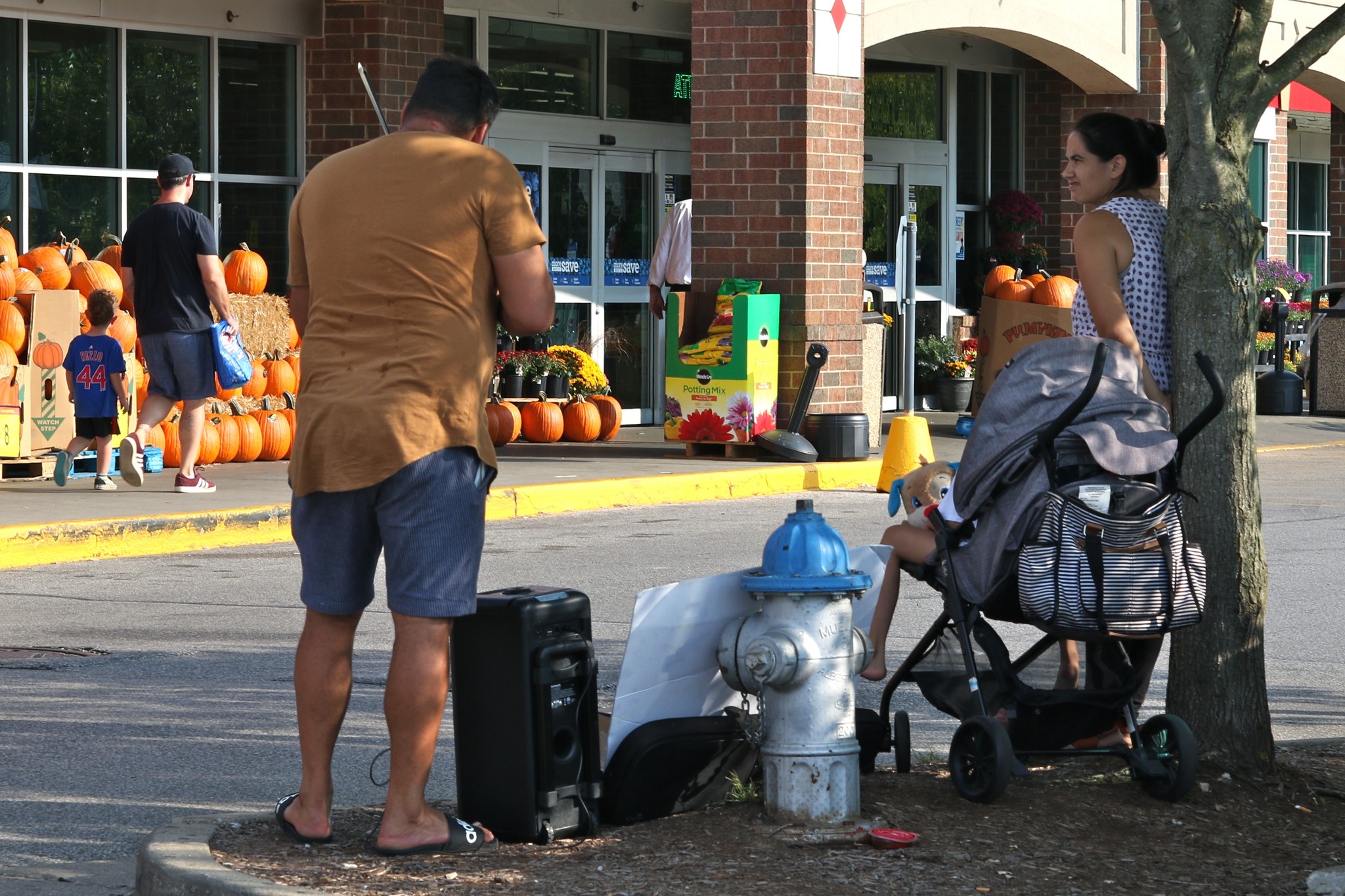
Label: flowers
xmin=957 ymin=335 xmax=982 ymax=359
xmin=1254 ymin=331 xmax=1286 ymax=351
xmin=545 ymin=338 xmax=612 ymax=393
xmin=1250 ymin=250 xmax=1323 ymax=287
xmin=883 ymin=310 xmax=896 ymax=336
xmin=985 ymin=186 xmax=1044 ymax=234
xmin=492 ymin=345 xmax=569 ymax=379
xmin=985 ymin=243 xmax=1049 ymax=265
xmin=1260 ymin=300 xmax=1330 ymax=322
xmin=942 ymin=360 xmax=974 ymax=379
xmin=1283 ymin=348 xmax=1303 ymax=374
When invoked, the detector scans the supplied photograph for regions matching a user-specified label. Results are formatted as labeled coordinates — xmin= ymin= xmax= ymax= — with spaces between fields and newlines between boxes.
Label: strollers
xmin=854 ymin=338 xmax=1230 ymax=805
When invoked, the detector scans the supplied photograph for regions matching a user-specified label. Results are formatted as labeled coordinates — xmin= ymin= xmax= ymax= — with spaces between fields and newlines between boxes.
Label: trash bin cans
xmin=862 ymin=281 xmax=893 ymax=448
xmin=1310 ymin=281 xmax=1344 ymax=416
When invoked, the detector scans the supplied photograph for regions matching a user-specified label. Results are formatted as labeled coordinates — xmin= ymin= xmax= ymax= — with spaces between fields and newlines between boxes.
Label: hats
xmin=158 ymin=152 xmax=201 ymax=178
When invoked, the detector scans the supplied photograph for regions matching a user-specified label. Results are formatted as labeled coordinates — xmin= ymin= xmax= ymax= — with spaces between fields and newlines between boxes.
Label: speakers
xmin=454 ymin=584 xmax=604 ymax=848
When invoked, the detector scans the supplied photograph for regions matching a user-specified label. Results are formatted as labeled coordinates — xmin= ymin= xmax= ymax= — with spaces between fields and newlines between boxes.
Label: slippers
xmin=374 ymin=812 xmax=499 ymax=857
xmin=275 ymin=791 xmax=335 ymax=844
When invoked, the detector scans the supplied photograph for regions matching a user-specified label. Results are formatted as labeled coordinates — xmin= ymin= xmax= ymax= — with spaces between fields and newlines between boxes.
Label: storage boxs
xmin=27 ymin=290 xmax=83 ymax=458
xmin=113 ymin=340 xmax=144 ymax=456
xmin=968 ymin=292 xmax=1082 ymax=422
xmin=0 ymin=288 xmax=37 ymax=458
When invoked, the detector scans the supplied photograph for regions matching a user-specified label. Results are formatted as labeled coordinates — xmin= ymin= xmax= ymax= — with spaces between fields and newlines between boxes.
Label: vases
xmin=1263 ymin=319 xmax=1311 ymax=334
xmin=936 ymin=379 xmax=974 ymax=416
xmin=486 ymin=371 xmax=572 ymax=399
xmin=987 ymin=260 xmax=1047 ymax=281
xmin=1253 ymin=350 xmax=1276 ymax=364
xmin=1258 ymin=289 xmax=1305 ymax=304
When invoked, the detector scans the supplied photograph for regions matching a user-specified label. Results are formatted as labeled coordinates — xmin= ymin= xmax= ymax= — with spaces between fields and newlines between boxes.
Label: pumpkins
xmin=222 ymin=242 xmax=267 ymax=296
xmin=142 ymin=318 xmax=302 ymax=467
xmin=1032 ymin=269 xmax=1078 ymax=308
xmin=1026 ymin=274 xmax=1045 ymax=286
xmin=0 ymin=215 xmax=150 ymax=411
xmin=218 ymin=258 xmax=225 ymax=278
xmin=520 ymin=385 xmax=622 ymax=444
xmin=485 ymin=394 xmax=522 ymax=447
xmin=995 ymin=268 xmax=1035 ymax=303
xmin=984 ymin=265 xmax=1016 ymax=298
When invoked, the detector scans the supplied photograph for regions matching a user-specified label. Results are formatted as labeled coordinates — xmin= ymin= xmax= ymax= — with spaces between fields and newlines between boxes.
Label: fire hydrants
xmin=716 ymin=498 xmax=876 ymax=848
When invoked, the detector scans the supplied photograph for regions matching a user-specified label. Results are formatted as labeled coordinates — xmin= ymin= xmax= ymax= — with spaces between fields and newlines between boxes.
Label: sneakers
xmin=174 ymin=464 xmax=217 ymax=493
xmin=53 ymin=450 xmax=73 ymax=487
xmin=94 ymin=476 xmax=117 ymax=490
xmin=119 ymin=432 xmax=145 ymax=486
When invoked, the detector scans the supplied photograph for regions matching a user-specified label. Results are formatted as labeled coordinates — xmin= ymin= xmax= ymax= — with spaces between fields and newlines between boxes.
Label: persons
xmin=274 ymin=55 xmax=555 ymax=852
xmin=53 ymin=288 xmax=130 ymax=491
xmin=120 ymin=152 xmax=239 ymax=493
xmin=861 ymin=114 xmax=1166 ymax=752
xmin=647 ymin=199 xmax=693 ymax=319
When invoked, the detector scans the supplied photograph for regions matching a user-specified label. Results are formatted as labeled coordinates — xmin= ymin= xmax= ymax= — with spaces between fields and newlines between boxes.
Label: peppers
xmin=664 ymin=412 xmax=684 ymax=440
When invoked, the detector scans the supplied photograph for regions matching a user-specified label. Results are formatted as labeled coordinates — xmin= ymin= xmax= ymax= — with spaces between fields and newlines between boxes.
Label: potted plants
xmin=915 ymin=330 xmax=955 ymax=378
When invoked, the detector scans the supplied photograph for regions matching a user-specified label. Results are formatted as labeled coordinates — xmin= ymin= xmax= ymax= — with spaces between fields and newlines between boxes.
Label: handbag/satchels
xmin=212 ymin=314 xmax=253 ymax=389
xmin=1012 ymin=492 xmax=1207 ymax=636
